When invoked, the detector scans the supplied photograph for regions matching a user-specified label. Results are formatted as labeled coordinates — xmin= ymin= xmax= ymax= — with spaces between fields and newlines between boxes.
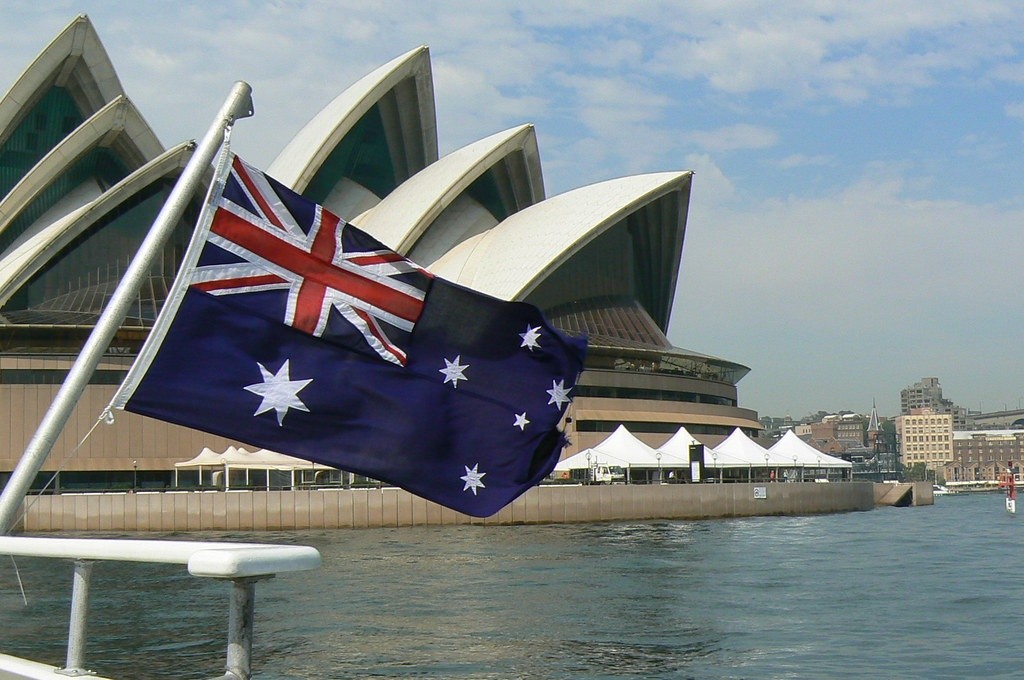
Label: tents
xmin=554 ymin=424 xmax=852 ymax=484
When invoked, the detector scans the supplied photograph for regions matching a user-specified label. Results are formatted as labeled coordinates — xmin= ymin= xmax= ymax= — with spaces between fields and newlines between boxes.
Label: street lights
xmin=792 ymin=455 xmax=799 ymax=483
xmin=132 ymin=461 xmax=137 ymax=493
xmin=712 ymin=452 xmax=717 ymax=483
xmin=584 ymin=450 xmax=592 ymax=486
xmin=764 ymin=453 xmax=769 ymax=483
xmin=220 ymin=458 xmax=226 ymax=492
xmin=816 ymin=456 xmax=822 ymax=484
xmin=655 ymin=452 xmax=662 ymax=484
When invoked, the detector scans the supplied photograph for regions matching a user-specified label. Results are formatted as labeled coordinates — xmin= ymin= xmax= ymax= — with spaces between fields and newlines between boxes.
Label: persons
xmin=783 ymin=469 xmax=788 ymax=483
xmin=770 ymin=470 xmax=775 ymax=483
xmin=669 ymin=471 xmax=674 ymax=484
xmin=561 ymin=471 xmax=568 ymax=479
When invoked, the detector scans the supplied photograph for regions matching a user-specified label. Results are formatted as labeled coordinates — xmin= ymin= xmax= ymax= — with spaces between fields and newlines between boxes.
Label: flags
xmin=109 ymin=145 xmax=589 ymax=518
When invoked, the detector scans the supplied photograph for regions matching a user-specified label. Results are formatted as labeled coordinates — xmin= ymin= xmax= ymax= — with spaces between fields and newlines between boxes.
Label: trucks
xmin=588 ymin=463 xmax=626 ymax=485
